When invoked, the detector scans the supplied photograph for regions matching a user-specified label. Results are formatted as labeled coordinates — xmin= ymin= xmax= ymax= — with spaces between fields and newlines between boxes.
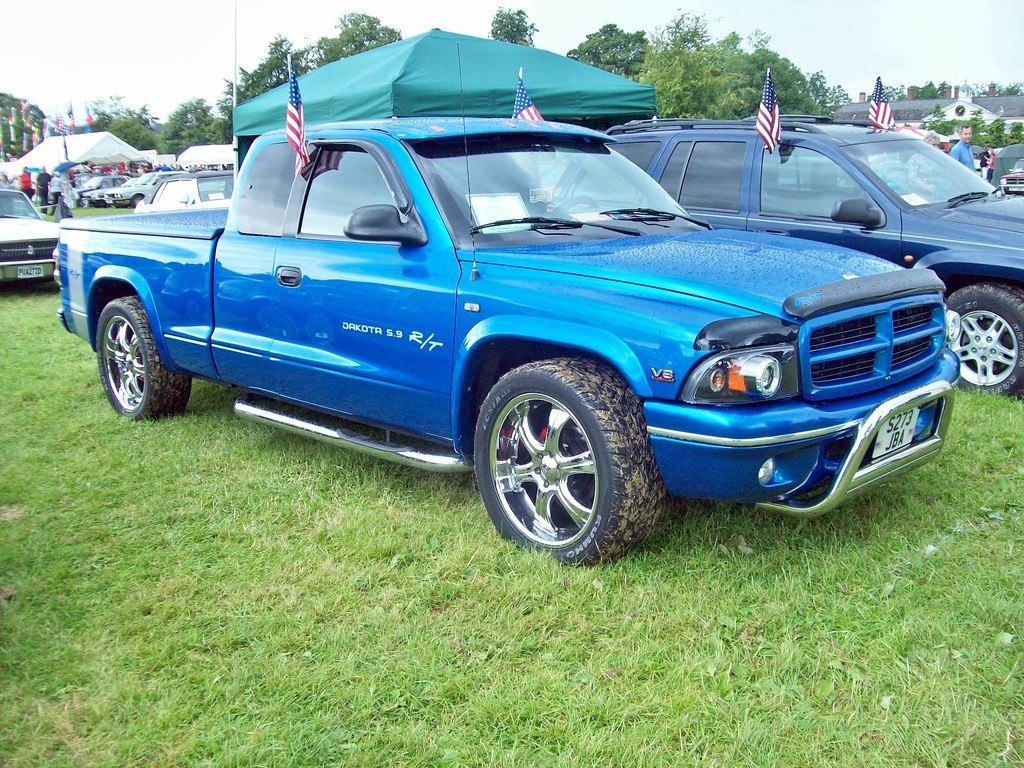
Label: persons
xmin=978 ymin=146 xmax=990 ymax=181
xmin=984 ymin=147 xmax=996 ymax=182
xmin=906 ymin=129 xmax=942 ymax=193
xmin=949 ymin=123 xmax=976 ymax=175
xmin=0 ymin=160 xmax=211 ymax=217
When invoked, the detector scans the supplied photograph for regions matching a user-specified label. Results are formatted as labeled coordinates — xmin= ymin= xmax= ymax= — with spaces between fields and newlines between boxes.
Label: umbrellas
xmin=892 ymin=123 xmax=950 ymax=143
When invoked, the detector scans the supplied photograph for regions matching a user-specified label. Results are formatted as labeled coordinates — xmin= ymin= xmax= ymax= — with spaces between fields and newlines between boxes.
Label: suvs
xmin=1000 ymin=157 xmax=1024 ymax=194
xmin=603 ymin=114 xmax=1024 ymax=396
xmin=104 ymin=171 xmax=190 ymax=207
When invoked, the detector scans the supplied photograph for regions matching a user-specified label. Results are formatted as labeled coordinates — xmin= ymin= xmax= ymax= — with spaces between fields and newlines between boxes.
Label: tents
xmin=0 ymin=27 xmax=660 ymax=184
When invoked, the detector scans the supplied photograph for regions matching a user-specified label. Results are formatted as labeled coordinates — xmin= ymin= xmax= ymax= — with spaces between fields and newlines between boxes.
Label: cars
xmin=81 ymin=177 xmax=141 ymax=209
xmin=0 ymin=188 xmax=59 ymax=284
xmin=73 ymin=175 xmax=131 ymax=207
xmin=134 ymin=170 xmax=234 ymax=214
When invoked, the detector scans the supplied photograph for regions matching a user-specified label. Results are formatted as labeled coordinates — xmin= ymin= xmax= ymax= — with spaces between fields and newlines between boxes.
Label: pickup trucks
xmin=50 ymin=116 xmax=963 ymax=569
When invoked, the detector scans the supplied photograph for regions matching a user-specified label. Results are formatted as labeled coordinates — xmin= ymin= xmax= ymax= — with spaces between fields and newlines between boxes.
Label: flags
xmin=299 ymin=147 xmax=343 ymax=183
xmin=756 ymin=72 xmax=782 ymax=155
xmin=867 ymin=77 xmax=897 ymax=132
xmin=285 ymin=60 xmax=311 ymax=171
xmin=511 ymin=77 xmax=545 ymax=122
